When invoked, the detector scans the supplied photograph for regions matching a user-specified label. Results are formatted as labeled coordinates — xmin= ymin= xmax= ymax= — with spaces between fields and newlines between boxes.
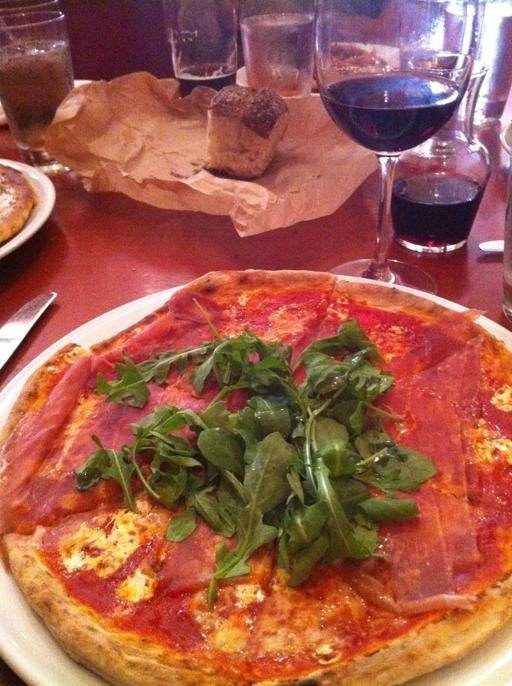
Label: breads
xmin=204 ymin=84 xmax=290 ymax=179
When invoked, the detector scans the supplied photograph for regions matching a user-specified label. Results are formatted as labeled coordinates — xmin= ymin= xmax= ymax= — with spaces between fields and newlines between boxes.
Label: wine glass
xmin=313 ymin=0 xmax=476 ymax=309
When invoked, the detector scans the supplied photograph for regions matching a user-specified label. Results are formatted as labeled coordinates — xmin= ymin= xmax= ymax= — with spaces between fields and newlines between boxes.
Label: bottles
xmin=387 ymin=51 xmax=493 ymax=259
xmin=163 ymin=0 xmax=314 ymax=104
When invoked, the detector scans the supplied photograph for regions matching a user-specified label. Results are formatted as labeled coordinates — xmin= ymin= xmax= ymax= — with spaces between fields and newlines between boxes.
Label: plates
xmin=1 ymin=160 xmax=63 ymax=266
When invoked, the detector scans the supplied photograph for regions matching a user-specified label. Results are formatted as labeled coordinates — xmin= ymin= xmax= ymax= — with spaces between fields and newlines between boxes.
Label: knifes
xmin=0 ymin=287 xmax=64 ymax=375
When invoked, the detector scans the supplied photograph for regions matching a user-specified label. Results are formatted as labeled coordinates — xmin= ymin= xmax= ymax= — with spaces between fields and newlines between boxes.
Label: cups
xmin=500 ymin=172 xmax=512 ymax=332
xmin=450 ymin=0 xmax=512 ymax=129
xmin=1 ymin=4 xmax=77 ymax=177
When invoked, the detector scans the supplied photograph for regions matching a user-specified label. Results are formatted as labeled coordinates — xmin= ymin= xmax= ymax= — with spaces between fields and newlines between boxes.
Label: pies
xmin=0 ymin=163 xmax=36 ymax=243
